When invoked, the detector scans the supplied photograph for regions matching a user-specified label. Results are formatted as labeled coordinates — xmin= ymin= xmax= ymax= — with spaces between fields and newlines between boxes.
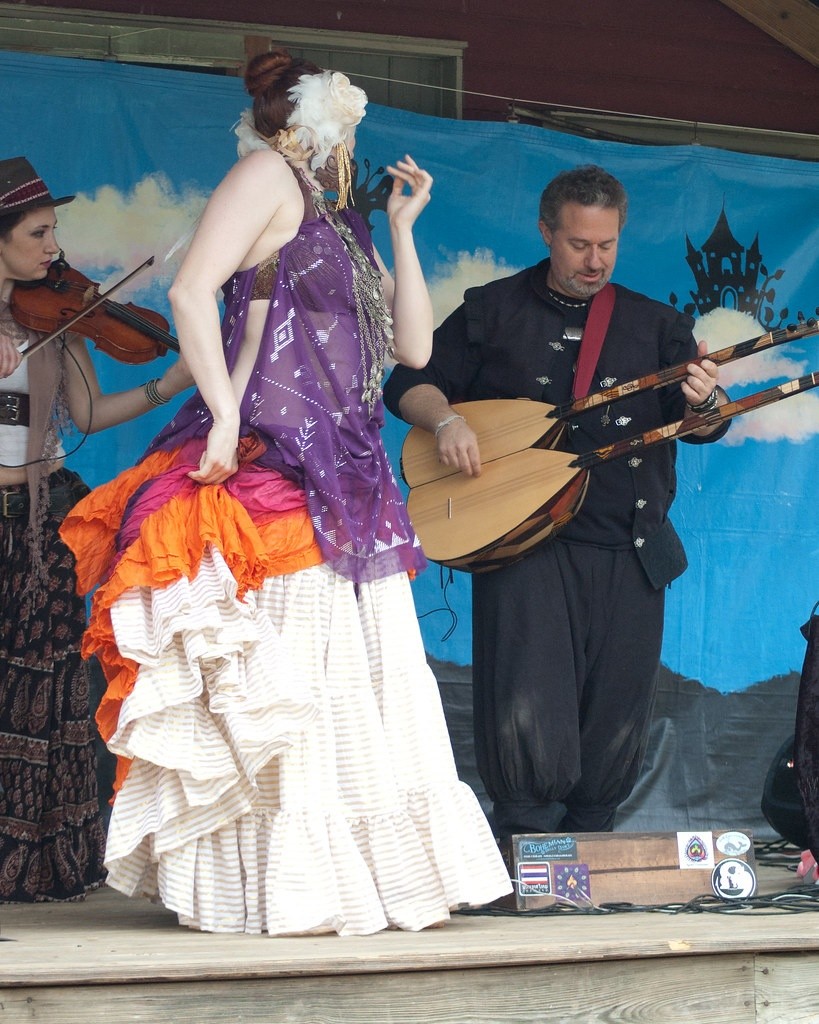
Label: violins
xmin=10 ymin=258 xmax=179 ymax=366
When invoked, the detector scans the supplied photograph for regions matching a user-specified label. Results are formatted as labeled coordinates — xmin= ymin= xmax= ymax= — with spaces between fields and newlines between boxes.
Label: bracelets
xmin=435 ymin=415 xmax=464 ymax=437
xmin=145 ymin=377 xmax=171 ymax=406
xmin=688 ymin=391 xmax=720 ymax=414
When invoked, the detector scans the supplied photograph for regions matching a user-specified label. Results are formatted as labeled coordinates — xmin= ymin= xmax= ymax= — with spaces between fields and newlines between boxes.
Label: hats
xmin=0 ymin=157 xmax=75 ymax=216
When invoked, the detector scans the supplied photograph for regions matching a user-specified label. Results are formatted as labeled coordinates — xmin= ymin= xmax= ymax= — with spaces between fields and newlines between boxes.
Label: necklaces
xmin=313 ymin=201 xmax=397 ymax=428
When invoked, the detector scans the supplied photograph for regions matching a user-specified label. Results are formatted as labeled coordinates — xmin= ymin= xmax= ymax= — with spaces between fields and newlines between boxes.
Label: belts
xmin=1 ymin=481 xmax=72 ymax=517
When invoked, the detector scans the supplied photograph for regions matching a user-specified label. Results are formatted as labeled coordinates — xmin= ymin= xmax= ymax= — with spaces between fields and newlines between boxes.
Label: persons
xmin=0 ymin=158 xmax=196 ymax=905
xmin=60 ymin=53 xmax=513 ymax=940
xmin=383 ymin=165 xmax=731 ymax=912
